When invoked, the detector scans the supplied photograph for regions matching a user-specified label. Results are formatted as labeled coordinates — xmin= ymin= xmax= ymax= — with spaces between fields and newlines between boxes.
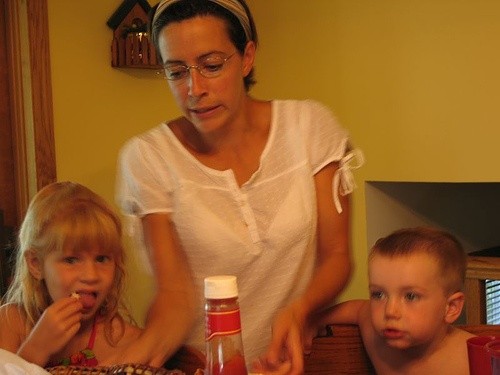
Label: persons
xmin=300 ymin=227 xmax=481 ymax=375
xmin=97 ymin=0 xmax=366 ymax=375
xmin=0 ymin=180 xmax=150 ymax=375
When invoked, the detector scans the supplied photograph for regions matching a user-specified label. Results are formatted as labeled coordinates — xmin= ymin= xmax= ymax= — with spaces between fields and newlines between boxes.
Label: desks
xmin=464 ymin=246 xmax=500 ymax=325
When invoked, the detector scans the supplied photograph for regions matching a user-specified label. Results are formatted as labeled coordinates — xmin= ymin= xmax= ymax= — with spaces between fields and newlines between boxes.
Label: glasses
xmin=156 ymin=50 xmax=240 ymax=81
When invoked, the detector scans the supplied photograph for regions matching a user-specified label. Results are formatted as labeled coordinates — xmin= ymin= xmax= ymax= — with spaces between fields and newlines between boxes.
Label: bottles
xmin=200 ymin=276 xmax=248 ymax=375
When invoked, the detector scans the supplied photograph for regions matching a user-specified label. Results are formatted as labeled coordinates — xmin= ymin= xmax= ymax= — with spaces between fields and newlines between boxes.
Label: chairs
xmin=303 ymin=324 xmax=500 ymax=375
